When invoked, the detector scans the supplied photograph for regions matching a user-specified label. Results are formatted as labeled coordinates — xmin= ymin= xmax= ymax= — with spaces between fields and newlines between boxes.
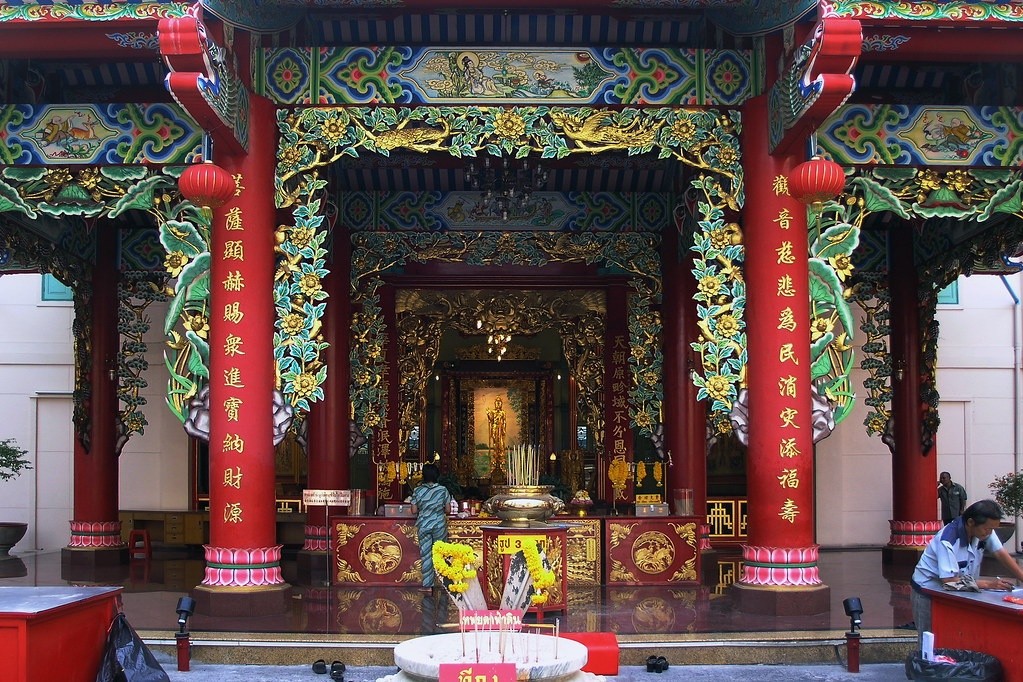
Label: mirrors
xmin=842 ymin=597 xmax=864 ymax=637
xmin=175 ymin=596 xmax=197 ymax=637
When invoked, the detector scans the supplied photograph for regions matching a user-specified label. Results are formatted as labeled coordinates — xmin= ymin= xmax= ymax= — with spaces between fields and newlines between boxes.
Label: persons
xmin=937 ymin=472 xmax=968 ymax=526
xmin=487 ymin=395 xmax=508 ymax=474
xmin=908 ymin=500 xmax=1023 ymax=653
xmin=411 ymin=464 xmax=451 ymax=591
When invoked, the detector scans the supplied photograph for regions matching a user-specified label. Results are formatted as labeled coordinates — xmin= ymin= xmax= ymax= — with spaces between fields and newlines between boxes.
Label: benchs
xmin=542 ymin=632 xmax=617 ymax=675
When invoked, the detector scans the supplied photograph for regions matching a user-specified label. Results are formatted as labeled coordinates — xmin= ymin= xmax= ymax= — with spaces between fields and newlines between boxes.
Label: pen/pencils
xmin=996 ymin=576 xmax=1016 ymax=588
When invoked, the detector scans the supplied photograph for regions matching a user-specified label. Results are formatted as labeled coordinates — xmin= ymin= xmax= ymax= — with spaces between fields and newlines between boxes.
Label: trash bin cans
xmin=907 ymin=649 xmax=1000 ymax=682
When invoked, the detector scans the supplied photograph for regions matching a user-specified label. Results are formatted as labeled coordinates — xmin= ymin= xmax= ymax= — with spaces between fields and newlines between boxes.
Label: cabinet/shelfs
xmin=117 ymin=510 xmax=308 ymax=545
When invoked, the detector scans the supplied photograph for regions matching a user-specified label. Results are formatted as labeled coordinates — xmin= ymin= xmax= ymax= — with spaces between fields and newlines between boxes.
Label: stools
xmin=128 ymin=529 xmax=153 ymax=559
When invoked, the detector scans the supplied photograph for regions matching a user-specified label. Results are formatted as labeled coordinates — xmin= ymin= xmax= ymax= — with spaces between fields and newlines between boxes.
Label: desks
xmin=0 ymin=586 xmax=124 ymax=682
xmin=919 ymin=587 xmax=1023 ymax=682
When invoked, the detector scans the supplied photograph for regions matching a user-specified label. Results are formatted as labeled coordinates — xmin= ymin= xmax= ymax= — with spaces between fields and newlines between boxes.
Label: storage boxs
xmin=385 ymin=504 xmax=417 ymax=518
xmin=633 ymin=504 xmax=669 ymax=517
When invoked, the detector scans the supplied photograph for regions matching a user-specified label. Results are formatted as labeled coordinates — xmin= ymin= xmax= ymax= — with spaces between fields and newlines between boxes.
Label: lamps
xmin=463 ymin=154 xmax=552 ymax=220
